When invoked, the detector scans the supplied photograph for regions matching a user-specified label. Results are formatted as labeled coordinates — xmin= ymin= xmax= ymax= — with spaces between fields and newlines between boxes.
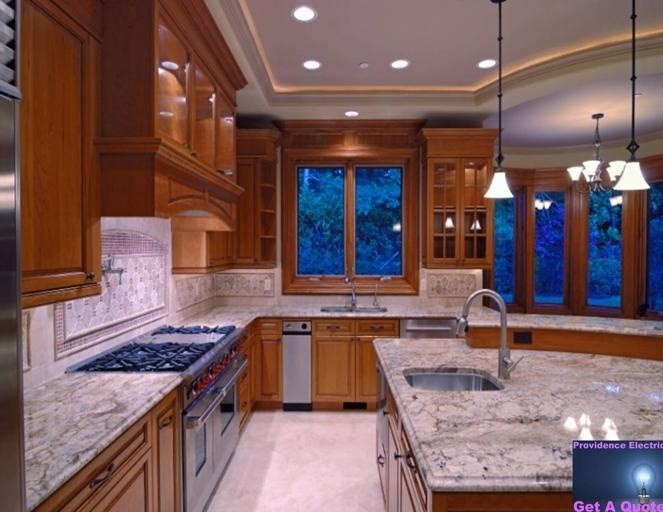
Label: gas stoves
xmin=66 ymin=324 xmax=246 ymax=412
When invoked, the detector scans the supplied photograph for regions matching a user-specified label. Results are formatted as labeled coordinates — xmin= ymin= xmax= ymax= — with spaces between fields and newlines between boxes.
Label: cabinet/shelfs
xmin=232 ymin=319 xmax=254 ymax=429
xmin=371 ymin=374 xmax=575 ymax=512
xmin=27 ymin=412 xmax=153 ymax=512
xmin=312 ymin=320 xmax=399 ymax=410
xmin=252 ymin=316 xmax=282 ymax=410
xmin=18 ymin=0 xmax=104 ymax=314
xmin=91 ymin=0 xmax=246 ymax=233
xmin=227 ymin=129 xmax=280 ymax=268
xmin=419 ymin=125 xmax=501 ymax=270
xmin=170 ymin=220 xmax=233 ymax=274
xmin=150 ymin=387 xmax=181 ymax=512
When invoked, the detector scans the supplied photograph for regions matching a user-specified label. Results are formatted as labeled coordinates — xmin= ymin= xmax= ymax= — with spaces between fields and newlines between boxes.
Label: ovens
xmin=181 ymin=355 xmax=248 ymax=512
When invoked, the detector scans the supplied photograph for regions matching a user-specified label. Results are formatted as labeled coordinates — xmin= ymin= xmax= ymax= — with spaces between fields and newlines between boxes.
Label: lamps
xmin=612 ymin=0 xmax=650 ymax=192
xmin=470 ymin=216 xmax=482 ymax=230
xmin=564 ymin=110 xmax=627 ymax=194
xmin=483 ymin=0 xmax=515 ymax=200
xmin=444 ymin=213 xmax=454 ymax=230
xmin=533 ymin=191 xmax=556 ymax=212
xmin=608 ymin=195 xmax=622 ymax=208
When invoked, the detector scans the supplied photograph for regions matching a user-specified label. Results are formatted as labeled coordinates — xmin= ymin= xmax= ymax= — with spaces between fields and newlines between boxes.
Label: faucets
xmin=454 ymin=287 xmax=525 ymax=382
xmin=343 ymin=276 xmax=358 ymax=307
xmin=372 ymin=284 xmax=380 ymax=306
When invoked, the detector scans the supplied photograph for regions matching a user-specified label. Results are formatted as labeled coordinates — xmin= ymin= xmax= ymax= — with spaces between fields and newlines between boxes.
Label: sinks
xmin=399 ymin=364 xmax=506 ymax=393
xmin=319 ymin=304 xmax=389 ymax=314
